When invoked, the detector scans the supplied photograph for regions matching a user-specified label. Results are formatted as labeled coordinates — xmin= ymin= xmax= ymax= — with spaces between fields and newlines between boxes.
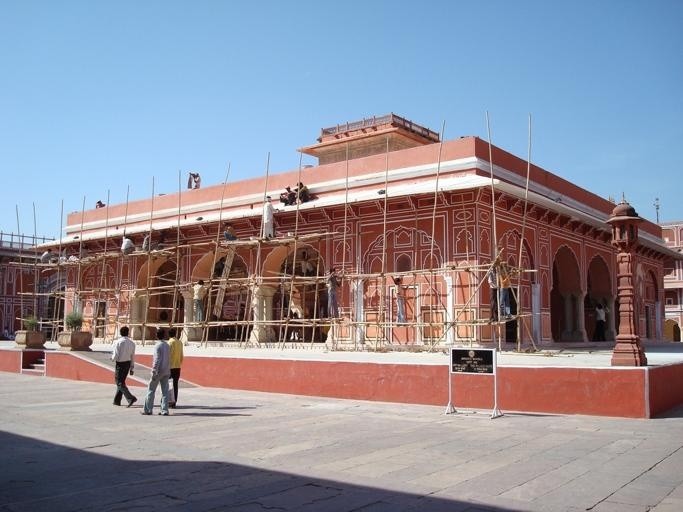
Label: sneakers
xmin=110 ymin=398 xmax=177 ymax=416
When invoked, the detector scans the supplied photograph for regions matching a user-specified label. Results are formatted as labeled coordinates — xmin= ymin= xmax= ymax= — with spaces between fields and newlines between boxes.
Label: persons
xmin=495 ymin=264 xmax=515 ymax=321
xmin=188 ymin=170 xmax=202 ymax=189
xmin=323 ymin=268 xmax=342 ymax=319
xmin=94 ymin=200 xmax=105 ymax=210
xmin=192 ymin=279 xmax=213 ymax=323
xmin=277 ymin=184 xmax=295 ymax=206
xmin=138 ymin=328 xmax=171 ymax=417
xmin=106 ymin=325 xmax=138 ymax=409
xmin=392 ymin=277 xmax=408 ymax=328
xmin=165 ymin=327 xmax=184 ymax=410
xmin=118 ymin=234 xmax=136 ymax=255
xmin=213 ymin=256 xmax=226 ymax=278
xmin=592 ymin=303 xmax=607 ymax=343
xmin=141 ymin=232 xmax=154 ymax=253
xmin=485 ymin=267 xmax=499 ymax=325
xmin=292 ymin=182 xmax=311 ymax=203
xmin=221 ymin=221 xmax=239 ymax=240
xmin=300 ymin=251 xmax=315 ymax=277
xmin=39 ymin=247 xmax=53 ymax=264
xmin=291 ymin=311 xmax=300 ymax=341
xmin=260 ymin=195 xmax=276 ymax=240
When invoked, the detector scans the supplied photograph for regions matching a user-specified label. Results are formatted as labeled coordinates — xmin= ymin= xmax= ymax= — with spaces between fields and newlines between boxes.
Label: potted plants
xmin=15 ymin=315 xmax=47 ymax=349
xmin=56 ymin=312 xmax=93 ymax=352
